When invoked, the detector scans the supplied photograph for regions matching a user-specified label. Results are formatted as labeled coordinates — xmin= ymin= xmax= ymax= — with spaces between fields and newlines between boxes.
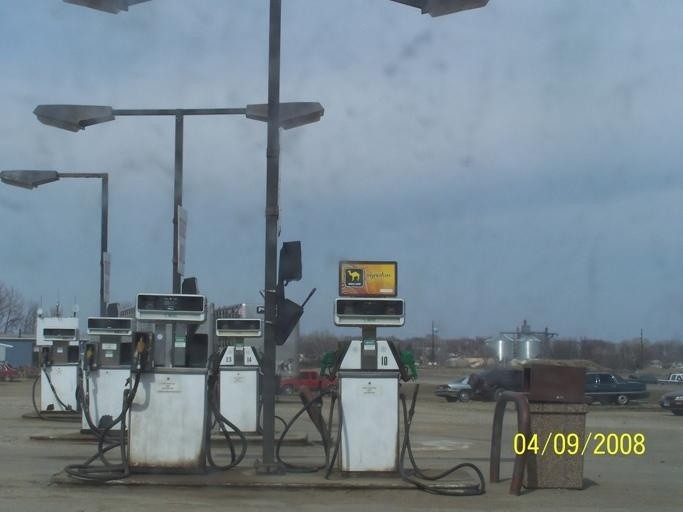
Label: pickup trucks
xmin=280 ymin=370 xmax=337 ymax=394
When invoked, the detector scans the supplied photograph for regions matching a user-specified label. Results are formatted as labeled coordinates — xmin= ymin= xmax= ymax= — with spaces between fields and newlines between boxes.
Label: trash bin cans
xmin=521 ymin=363 xmax=588 ymax=490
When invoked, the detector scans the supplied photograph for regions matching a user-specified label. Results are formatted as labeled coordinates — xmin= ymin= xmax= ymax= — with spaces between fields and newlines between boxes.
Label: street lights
xmin=0 ymin=167 xmax=110 ymax=319
xmin=60 ymin=1 xmax=487 ymax=474
xmin=30 ymin=100 xmax=324 ymax=292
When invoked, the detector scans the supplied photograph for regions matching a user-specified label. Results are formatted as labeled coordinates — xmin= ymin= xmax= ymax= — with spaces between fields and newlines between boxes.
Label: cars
xmin=436 ymin=364 xmax=683 ymax=416
xmin=0 ymin=360 xmax=20 ymax=382
